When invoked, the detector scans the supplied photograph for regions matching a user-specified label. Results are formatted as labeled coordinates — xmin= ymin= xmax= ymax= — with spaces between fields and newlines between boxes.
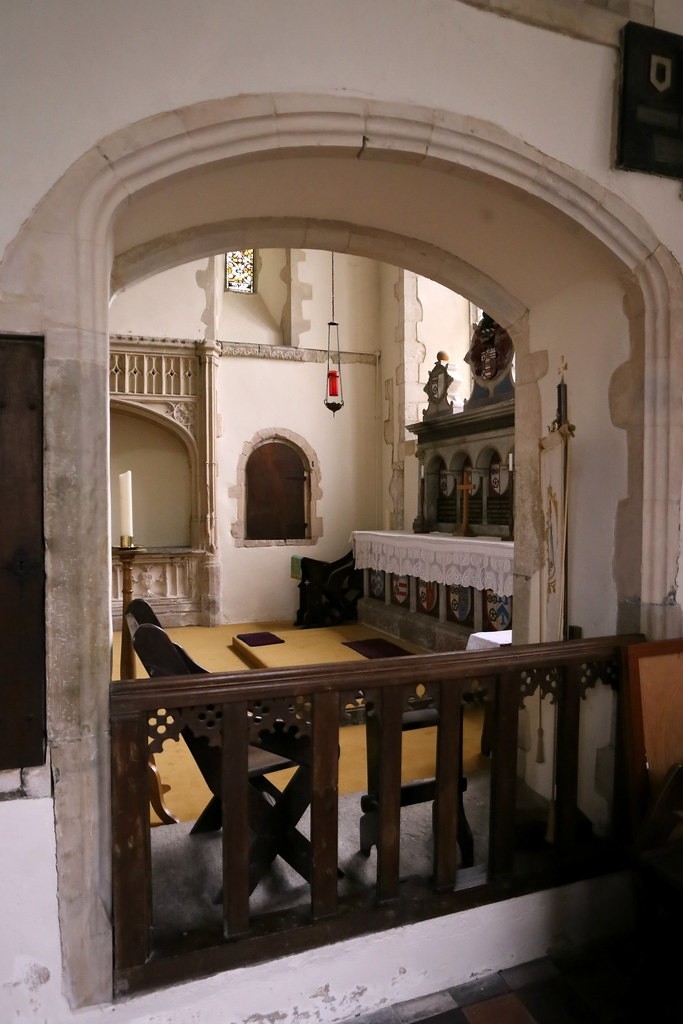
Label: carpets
xmin=237 ymin=632 xmax=285 ymax=647
xmin=341 ymin=639 xmax=414 ymax=659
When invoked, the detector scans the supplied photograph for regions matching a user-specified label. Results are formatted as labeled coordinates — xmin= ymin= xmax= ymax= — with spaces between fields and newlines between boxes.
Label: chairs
xmin=124 ymin=598 xmax=344 ymax=901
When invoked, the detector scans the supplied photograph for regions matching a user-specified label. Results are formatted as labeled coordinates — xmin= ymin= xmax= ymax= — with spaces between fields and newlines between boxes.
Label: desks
xmin=350 ymin=530 xmax=514 ymax=632
xmin=466 ymin=629 xmax=512 ymax=756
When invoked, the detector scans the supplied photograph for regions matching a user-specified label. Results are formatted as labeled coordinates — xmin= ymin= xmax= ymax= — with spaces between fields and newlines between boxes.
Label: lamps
xmin=325 ymin=252 xmax=344 ymax=417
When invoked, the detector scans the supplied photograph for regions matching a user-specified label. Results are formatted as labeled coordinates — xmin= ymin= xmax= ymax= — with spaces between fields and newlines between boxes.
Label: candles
xmin=421 ymin=465 xmax=424 ymax=478
xmin=118 ymin=471 xmax=133 ymax=538
xmin=509 ymin=453 xmax=513 ymax=471
